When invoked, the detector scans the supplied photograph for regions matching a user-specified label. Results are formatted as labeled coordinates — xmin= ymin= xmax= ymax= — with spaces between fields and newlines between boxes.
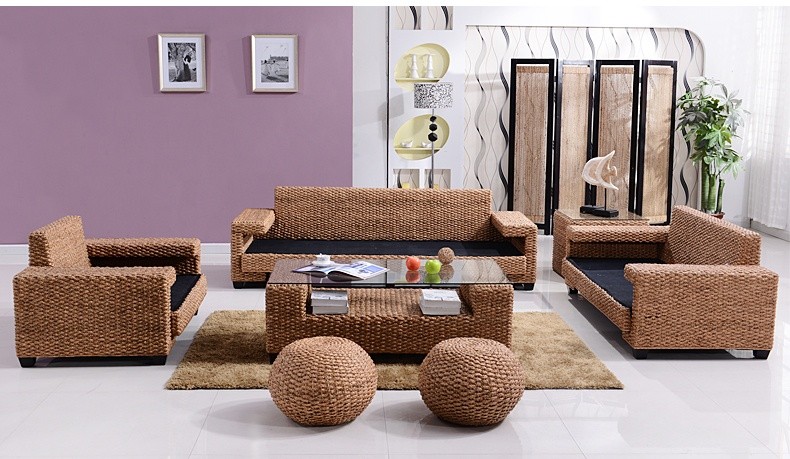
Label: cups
xmin=316 ymin=255 xmax=330 ymax=261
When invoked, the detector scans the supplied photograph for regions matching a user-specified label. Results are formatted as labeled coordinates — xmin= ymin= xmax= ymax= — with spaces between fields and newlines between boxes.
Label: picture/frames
xmin=251 ymin=34 xmax=299 ymax=92
xmin=157 ymin=32 xmax=207 ymax=92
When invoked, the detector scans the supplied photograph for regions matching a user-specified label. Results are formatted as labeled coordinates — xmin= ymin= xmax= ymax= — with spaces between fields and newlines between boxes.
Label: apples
xmin=406 ymin=255 xmax=420 ymax=270
xmin=406 ymin=270 xmax=420 ymax=282
xmin=425 ymin=259 xmax=441 ymax=274
xmin=424 ymin=273 xmax=441 ymax=283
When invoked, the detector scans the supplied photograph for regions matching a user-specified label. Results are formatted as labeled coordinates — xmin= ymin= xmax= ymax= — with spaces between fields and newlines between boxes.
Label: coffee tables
xmin=264 ymin=257 xmax=514 ymax=364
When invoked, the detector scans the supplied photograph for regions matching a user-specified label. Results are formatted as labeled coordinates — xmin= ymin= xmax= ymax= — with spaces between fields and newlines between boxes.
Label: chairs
xmin=11 ymin=215 xmax=208 ymax=366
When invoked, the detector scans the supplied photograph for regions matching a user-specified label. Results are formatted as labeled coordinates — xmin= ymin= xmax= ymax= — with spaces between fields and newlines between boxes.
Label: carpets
xmin=166 ymin=309 xmax=623 ymax=391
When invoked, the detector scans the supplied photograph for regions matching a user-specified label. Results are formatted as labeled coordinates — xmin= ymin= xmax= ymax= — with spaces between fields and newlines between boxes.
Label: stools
xmin=416 ymin=337 xmax=525 ymax=429
xmin=270 ymin=335 xmax=378 ymax=425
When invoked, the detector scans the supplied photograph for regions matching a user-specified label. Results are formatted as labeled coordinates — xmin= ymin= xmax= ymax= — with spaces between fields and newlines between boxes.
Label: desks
xmin=553 ymin=209 xmax=649 ymax=280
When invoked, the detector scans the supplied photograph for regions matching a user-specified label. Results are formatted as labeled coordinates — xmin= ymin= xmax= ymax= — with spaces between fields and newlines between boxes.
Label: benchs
xmin=230 ymin=186 xmax=537 ymax=290
xmin=560 ymin=207 xmax=779 ymax=359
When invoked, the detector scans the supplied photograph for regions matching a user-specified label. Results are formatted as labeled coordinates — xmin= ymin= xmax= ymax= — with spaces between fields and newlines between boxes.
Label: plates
xmin=312 ymin=261 xmax=335 ymax=267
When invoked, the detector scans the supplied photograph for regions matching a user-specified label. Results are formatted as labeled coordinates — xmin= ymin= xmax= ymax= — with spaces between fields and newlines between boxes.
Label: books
xmin=310 ymin=290 xmax=349 ymax=314
xmin=420 ymin=289 xmax=462 ymax=315
xmin=291 ymin=260 xmax=389 ymax=279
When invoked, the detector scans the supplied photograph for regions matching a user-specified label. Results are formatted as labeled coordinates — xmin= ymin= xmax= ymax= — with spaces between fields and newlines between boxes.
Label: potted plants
xmin=675 ymin=79 xmax=750 ymax=219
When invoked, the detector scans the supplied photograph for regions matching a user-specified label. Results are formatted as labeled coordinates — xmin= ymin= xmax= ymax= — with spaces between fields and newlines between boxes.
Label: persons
xmin=170 ymin=45 xmax=193 ymax=82
